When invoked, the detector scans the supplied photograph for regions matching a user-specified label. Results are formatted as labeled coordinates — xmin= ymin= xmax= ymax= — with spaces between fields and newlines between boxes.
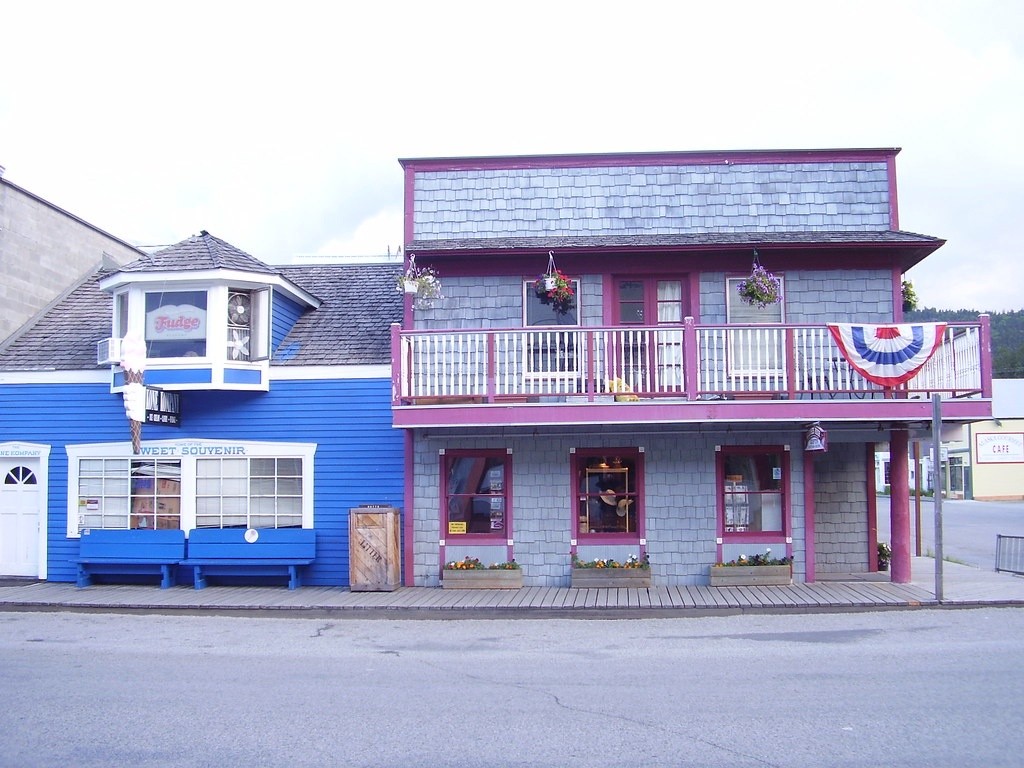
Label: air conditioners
xmin=97 ymin=338 xmax=122 ymax=366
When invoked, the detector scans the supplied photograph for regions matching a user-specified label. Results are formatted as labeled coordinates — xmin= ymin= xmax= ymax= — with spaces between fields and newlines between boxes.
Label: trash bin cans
xmin=348 ymin=504 xmax=402 ymax=591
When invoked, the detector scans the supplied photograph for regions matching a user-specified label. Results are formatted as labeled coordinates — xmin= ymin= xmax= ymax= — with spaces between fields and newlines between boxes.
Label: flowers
xmin=395 ymin=264 xmax=445 ymax=311
xmin=443 ymin=555 xmax=519 ymax=570
xmin=901 ymin=280 xmax=918 ymax=313
xmin=714 ymin=547 xmax=794 ymax=567
xmin=568 ymin=551 xmax=651 ymax=570
xmin=736 ymin=264 xmax=783 ymax=308
xmin=877 ymin=542 xmax=891 ymax=566
xmin=533 ymin=274 xmax=575 ymax=308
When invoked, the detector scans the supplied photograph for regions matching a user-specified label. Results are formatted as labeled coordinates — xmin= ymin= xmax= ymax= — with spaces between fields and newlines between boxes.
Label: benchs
xmin=67 ymin=528 xmax=185 ymax=589
xmin=179 ymin=529 xmax=316 ymax=590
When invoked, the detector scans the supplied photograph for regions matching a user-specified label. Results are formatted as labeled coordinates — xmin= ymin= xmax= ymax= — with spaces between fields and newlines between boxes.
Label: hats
xmin=616 ymin=499 xmax=633 ymax=517
xmin=599 ymin=489 xmax=617 ymax=506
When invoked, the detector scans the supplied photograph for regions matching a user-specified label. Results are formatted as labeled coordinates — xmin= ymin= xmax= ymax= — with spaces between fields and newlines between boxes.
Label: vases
xmin=571 ymin=568 xmax=651 ymax=588
xmin=902 ymin=300 xmax=913 ymax=313
xmin=544 ymin=278 xmax=558 ymax=291
xmin=402 ymin=281 xmax=419 ymax=294
xmin=879 ymin=561 xmax=888 ymax=570
xmin=709 ymin=565 xmax=791 ymax=585
xmin=442 ymin=570 xmax=522 ymax=589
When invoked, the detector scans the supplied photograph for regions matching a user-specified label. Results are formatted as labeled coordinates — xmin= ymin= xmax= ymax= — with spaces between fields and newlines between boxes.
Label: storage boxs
xmin=129 ymin=478 xmax=180 ymax=529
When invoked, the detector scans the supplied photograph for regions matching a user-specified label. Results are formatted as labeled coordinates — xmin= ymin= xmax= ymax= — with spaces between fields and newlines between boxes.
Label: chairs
xmin=792 ymin=347 xmax=833 ymax=400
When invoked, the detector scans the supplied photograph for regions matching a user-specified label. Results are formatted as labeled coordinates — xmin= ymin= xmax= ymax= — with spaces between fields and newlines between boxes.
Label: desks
xmin=827 ymin=357 xmax=868 ymax=399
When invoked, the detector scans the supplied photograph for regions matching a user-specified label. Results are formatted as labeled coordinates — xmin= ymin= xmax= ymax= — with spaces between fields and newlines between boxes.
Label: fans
xmin=228 ymin=293 xmax=250 ymax=326
